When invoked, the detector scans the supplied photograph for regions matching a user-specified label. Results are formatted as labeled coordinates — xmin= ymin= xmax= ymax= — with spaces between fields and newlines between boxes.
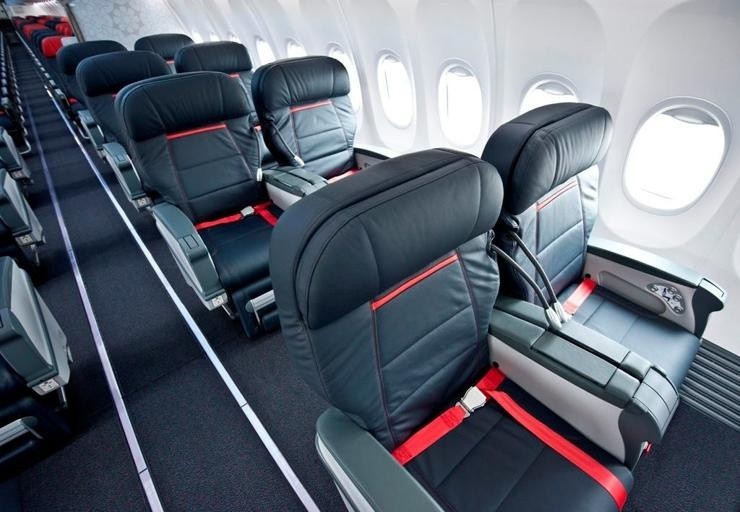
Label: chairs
xmin=251 ymin=55 xmax=392 ymax=189
xmin=12 ymin=15 xmax=73 ymax=58
xmin=76 ymin=51 xmax=175 ymax=213
xmin=134 ymin=33 xmax=196 ymax=74
xmin=270 ymin=149 xmax=671 ymax=512
xmin=55 ymin=40 xmax=128 ymax=164
xmin=174 ymin=41 xmax=280 ymax=170
xmin=479 ymin=102 xmax=724 ymax=456
xmin=115 ymin=71 xmax=319 ymax=340
